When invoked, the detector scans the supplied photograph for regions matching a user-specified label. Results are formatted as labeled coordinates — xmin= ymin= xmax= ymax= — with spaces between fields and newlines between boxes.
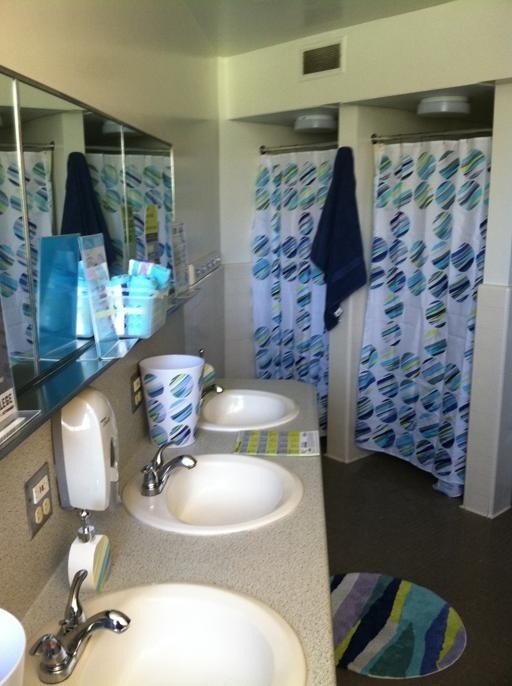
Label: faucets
xmin=203 ymin=384 xmax=225 ymax=398
xmin=29 ymin=573 xmax=130 ymax=682
xmin=132 ymin=442 xmax=196 ymax=494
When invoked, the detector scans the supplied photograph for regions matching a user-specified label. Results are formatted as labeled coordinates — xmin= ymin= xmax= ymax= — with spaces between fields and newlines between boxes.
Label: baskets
xmin=121 ymin=286 xmax=169 ymax=338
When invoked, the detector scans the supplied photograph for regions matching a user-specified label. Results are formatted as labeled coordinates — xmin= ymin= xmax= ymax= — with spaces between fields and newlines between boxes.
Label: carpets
xmin=328 ymin=572 xmax=468 ymax=681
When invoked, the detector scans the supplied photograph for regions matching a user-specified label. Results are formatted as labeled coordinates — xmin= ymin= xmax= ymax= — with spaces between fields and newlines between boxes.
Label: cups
xmin=137 ymin=353 xmax=208 ymax=450
xmin=0 ymin=607 xmax=29 ymax=686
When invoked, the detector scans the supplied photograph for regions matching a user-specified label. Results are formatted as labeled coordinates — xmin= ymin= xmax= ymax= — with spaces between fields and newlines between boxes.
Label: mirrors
xmin=1 ymin=64 xmax=177 ymax=399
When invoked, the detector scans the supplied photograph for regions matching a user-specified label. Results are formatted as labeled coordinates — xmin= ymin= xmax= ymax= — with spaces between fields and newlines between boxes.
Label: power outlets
xmin=24 ymin=461 xmax=54 ymax=541
xmin=131 ymin=372 xmax=143 ymax=413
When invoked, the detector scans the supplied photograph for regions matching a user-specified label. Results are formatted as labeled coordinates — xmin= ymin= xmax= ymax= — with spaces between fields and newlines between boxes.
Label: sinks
xmin=200 ymin=388 xmax=298 ymax=431
xmin=71 ymin=581 xmax=306 ymax=686
xmin=124 ymin=453 xmax=302 ymax=536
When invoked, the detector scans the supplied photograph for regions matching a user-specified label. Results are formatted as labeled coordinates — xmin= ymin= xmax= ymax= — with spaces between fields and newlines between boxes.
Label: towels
xmin=310 ymin=146 xmax=368 ymax=331
xmin=60 ymin=151 xmax=117 ymax=267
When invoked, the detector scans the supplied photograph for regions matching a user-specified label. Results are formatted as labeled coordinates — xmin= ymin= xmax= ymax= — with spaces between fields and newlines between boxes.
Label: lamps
xmin=102 ymin=119 xmax=134 ymax=134
xmin=417 ymin=96 xmax=471 ymax=117
xmin=295 ymin=114 xmax=337 ymax=133
